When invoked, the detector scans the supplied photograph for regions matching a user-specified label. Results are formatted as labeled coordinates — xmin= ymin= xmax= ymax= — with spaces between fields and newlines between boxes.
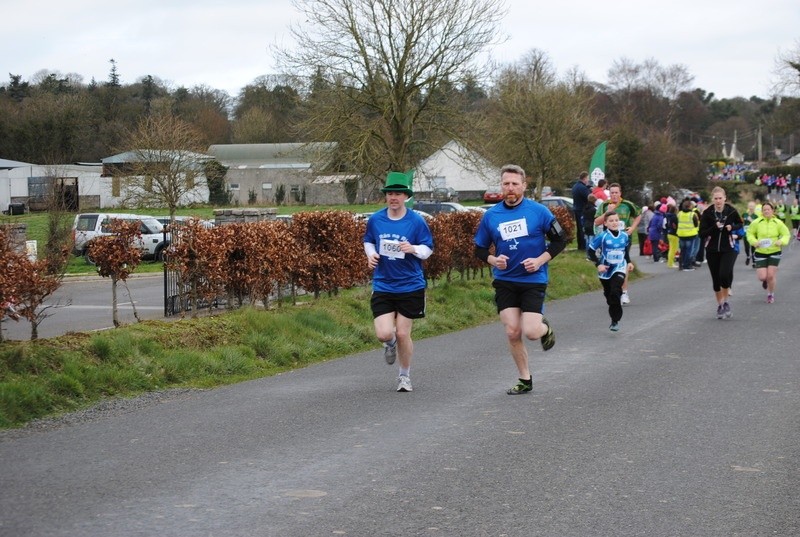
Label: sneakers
xmin=723 ymin=301 xmax=732 ymax=318
xmin=763 ymin=280 xmax=767 ymax=290
xmin=609 ymin=323 xmax=619 ymax=330
xmin=396 ymin=374 xmax=412 ymax=392
xmin=717 ymin=303 xmax=724 ymax=318
xmin=507 ymin=375 xmax=533 ymax=394
xmin=384 ymin=334 xmax=397 ymax=365
xmin=768 ymin=292 xmax=775 ymax=303
xmin=620 ymin=290 xmax=631 ymax=304
xmin=541 ymin=318 xmax=555 ymax=351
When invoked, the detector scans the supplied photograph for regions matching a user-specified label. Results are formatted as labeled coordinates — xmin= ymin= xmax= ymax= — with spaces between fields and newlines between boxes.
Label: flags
xmin=588 ymin=141 xmax=606 ymax=181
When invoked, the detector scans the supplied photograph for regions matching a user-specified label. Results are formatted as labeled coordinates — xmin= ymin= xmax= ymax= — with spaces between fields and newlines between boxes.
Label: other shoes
xmin=641 ymin=238 xmax=757 ymax=272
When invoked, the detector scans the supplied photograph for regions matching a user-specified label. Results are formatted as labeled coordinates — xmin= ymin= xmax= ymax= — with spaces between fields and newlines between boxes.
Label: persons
xmin=363 ymin=171 xmax=435 ymax=392
xmin=570 ymin=159 xmax=800 ymax=272
xmin=745 ymin=201 xmax=791 ymax=304
xmin=698 ymin=186 xmax=742 ymax=319
xmin=472 ymin=164 xmax=568 ymax=396
xmin=586 ymin=211 xmax=634 ymax=331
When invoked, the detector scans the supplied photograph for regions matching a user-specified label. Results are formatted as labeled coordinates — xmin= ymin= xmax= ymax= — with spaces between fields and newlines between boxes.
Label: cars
xmin=430 ymin=188 xmax=458 ymax=203
xmin=536 ymin=198 xmax=578 ymax=221
xmin=639 ymin=181 xmax=701 ymax=209
xmin=157 ymin=215 xmax=216 ymax=231
xmin=483 ymin=185 xmax=503 ymax=203
xmin=359 ymin=202 xmax=497 ymax=224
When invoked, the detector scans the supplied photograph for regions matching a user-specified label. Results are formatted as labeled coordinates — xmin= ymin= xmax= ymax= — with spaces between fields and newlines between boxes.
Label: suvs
xmin=69 ymin=213 xmax=179 ymax=266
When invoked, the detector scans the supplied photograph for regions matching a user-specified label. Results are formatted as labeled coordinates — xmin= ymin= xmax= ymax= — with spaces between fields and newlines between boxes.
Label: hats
xmin=381 ymin=172 xmax=413 ymax=197
